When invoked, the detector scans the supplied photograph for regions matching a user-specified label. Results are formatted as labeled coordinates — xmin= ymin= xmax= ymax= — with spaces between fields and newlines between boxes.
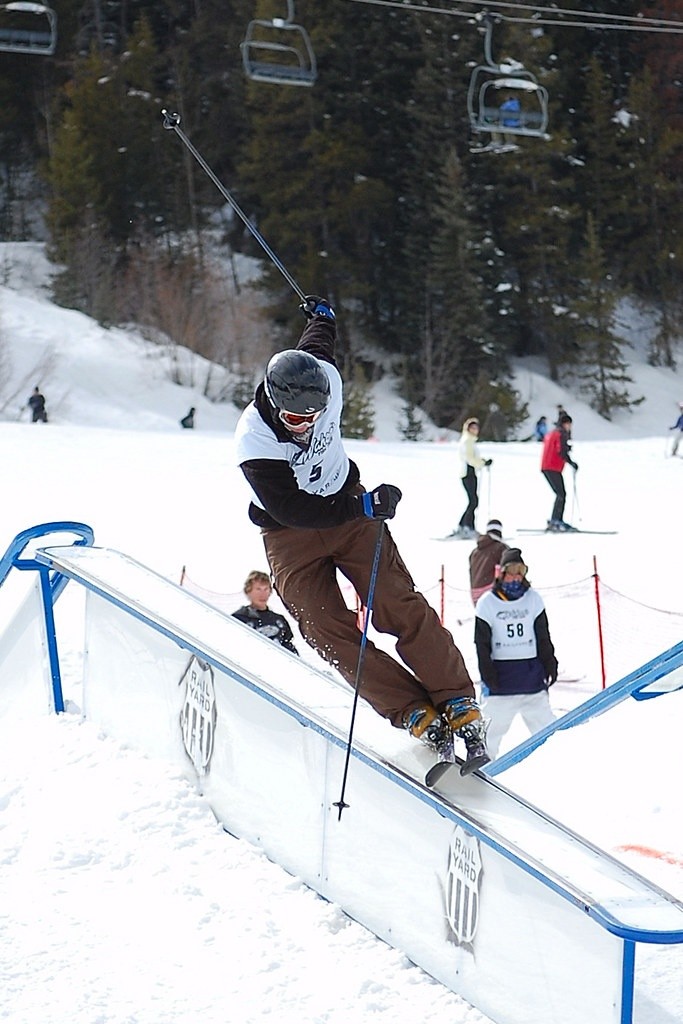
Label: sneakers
xmin=404 ymin=705 xmax=446 ymax=743
xmin=444 ymin=698 xmax=483 ymax=741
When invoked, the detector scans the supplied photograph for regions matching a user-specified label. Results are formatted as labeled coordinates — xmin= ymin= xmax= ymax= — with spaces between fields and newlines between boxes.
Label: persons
xmin=456 ymin=421 xmax=493 ymax=536
xmin=231 ymin=295 xmax=481 ymax=749
xmin=468 ymin=519 xmax=512 ymax=697
xmin=232 ymin=571 xmax=300 ymax=657
xmin=536 ymin=416 xmax=548 ymax=441
xmin=181 ymin=407 xmax=195 ymax=428
xmin=474 ymin=548 xmax=559 ymax=761
xmin=492 ymin=93 xmax=521 ymax=145
xmin=557 ymin=405 xmax=568 ymax=420
xmin=540 ymin=415 xmax=578 ymax=531
xmin=28 ymin=387 xmax=48 ymax=422
xmin=669 ymin=406 xmax=683 ymax=456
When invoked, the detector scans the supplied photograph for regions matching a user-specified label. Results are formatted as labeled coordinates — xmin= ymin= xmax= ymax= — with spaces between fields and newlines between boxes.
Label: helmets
xmin=266 ymin=348 xmax=330 ymax=415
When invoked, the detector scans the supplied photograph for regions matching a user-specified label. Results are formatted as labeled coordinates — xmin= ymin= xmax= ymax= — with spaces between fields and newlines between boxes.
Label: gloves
xmin=486 ymin=459 xmax=492 ymax=466
xmin=482 ymin=673 xmax=495 ymax=689
xmin=546 ymin=667 xmax=558 ymax=686
xmin=362 ymin=484 xmax=402 ymax=520
xmin=299 ymin=295 xmax=335 ymax=319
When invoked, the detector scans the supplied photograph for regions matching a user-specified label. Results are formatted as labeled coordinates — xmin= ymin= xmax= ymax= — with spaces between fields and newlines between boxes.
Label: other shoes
xmin=547 ymin=520 xmax=571 ymax=530
xmin=457 ymin=527 xmax=479 ymax=536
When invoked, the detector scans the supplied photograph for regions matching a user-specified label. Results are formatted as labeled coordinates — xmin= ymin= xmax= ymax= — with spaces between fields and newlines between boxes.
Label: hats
xmin=487 ymin=520 xmax=502 ymax=537
xmin=497 ymin=548 xmax=528 ymax=579
xmin=558 ymin=413 xmax=572 ymax=424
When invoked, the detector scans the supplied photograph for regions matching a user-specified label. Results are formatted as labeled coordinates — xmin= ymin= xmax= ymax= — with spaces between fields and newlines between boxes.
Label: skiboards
xmin=516 ymin=529 xmax=618 ymax=535
xmin=425 ymin=716 xmax=493 ymax=788
xmin=430 ymin=537 xmax=514 ymax=542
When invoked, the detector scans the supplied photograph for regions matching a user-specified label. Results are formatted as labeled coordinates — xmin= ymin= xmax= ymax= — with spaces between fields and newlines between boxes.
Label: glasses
xmin=505 ymin=562 xmax=526 ymax=575
xmin=278 ymin=404 xmax=325 ymax=427
